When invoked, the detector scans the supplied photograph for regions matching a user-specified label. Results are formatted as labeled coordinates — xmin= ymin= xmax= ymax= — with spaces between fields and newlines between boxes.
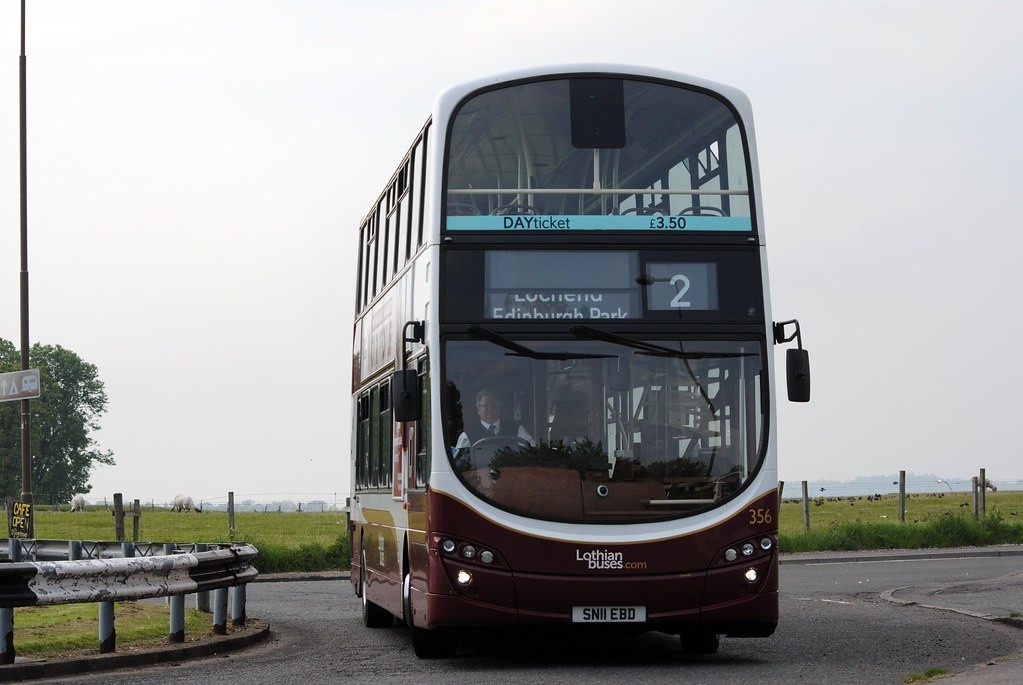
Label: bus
xmin=344 ymin=59 xmax=812 ymax=655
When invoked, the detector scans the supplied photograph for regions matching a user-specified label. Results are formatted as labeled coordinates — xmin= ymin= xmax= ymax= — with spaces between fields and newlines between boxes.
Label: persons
xmin=456 ymin=390 xmax=536 ymax=448
xmin=550 ymin=373 xmax=588 ymax=447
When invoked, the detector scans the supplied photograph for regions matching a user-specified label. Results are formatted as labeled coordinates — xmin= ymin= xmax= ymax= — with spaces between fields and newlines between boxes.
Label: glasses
xmin=477 ymin=402 xmax=500 ymax=409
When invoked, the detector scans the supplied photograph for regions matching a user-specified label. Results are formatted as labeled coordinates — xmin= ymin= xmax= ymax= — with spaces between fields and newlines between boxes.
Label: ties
xmin=489 ymin=424 xmax=496 ymax=433
